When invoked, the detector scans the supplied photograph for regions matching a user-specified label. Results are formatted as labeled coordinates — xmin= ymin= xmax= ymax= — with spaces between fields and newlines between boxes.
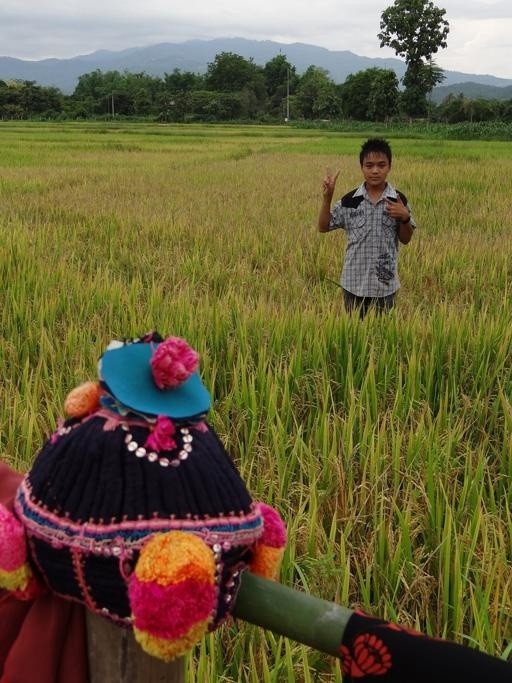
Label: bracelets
xmin=396 ymin=215 xmax=411 ymax=223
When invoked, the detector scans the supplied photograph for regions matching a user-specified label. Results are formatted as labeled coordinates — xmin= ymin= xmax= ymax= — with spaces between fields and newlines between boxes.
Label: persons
xmin=318 ymin=135 xmax=416 ymax=318
